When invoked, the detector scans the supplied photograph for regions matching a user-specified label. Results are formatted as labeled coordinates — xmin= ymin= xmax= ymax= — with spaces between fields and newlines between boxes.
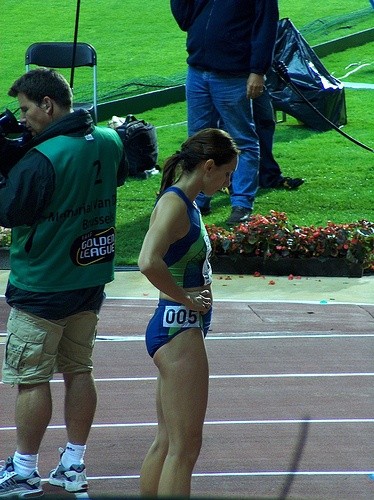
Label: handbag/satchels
xmin=116 ymin=114 xmax=158 ymax=176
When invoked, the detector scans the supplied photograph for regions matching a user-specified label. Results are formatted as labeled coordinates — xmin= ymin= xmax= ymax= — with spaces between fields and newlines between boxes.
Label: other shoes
xmin=199 ymin=204 xmax=212 ymax=214
xmin=272 ymin=176 xmax=303 ymax=189
xmin=226 ymin=206 xmax=250 ymax=224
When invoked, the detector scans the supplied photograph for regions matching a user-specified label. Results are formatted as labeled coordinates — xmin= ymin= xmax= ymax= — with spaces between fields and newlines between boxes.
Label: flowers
xmin=207 ymin=210 xmax=374 ymax=269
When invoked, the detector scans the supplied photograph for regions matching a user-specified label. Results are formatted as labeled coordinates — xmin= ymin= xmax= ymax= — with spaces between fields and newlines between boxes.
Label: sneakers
xmin=0 ymin=456 xmax=44 ymax=499
xmin=48 ymin=447 xmax=89 ymax=492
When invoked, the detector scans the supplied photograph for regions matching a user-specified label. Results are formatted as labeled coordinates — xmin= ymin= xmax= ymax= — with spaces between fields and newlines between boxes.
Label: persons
xmin=169 ymin=0 xmax=279 ymax=226
xmin=137 ymin=128 xmax=241 ymax=498
xmin=0 ymin=66 xmax=130 ymax=500
xmin=218 ymin=84 xmax=306 ymax=195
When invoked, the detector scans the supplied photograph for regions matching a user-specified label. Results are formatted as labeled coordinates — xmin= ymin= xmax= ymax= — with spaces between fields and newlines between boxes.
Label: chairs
xmin=25 ymin=42 xmax=98 ymax=128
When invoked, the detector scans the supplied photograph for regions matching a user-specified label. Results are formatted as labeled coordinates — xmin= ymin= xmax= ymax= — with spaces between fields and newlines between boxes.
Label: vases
xmin=211 ymin=254 xmax=374 ymax=279
